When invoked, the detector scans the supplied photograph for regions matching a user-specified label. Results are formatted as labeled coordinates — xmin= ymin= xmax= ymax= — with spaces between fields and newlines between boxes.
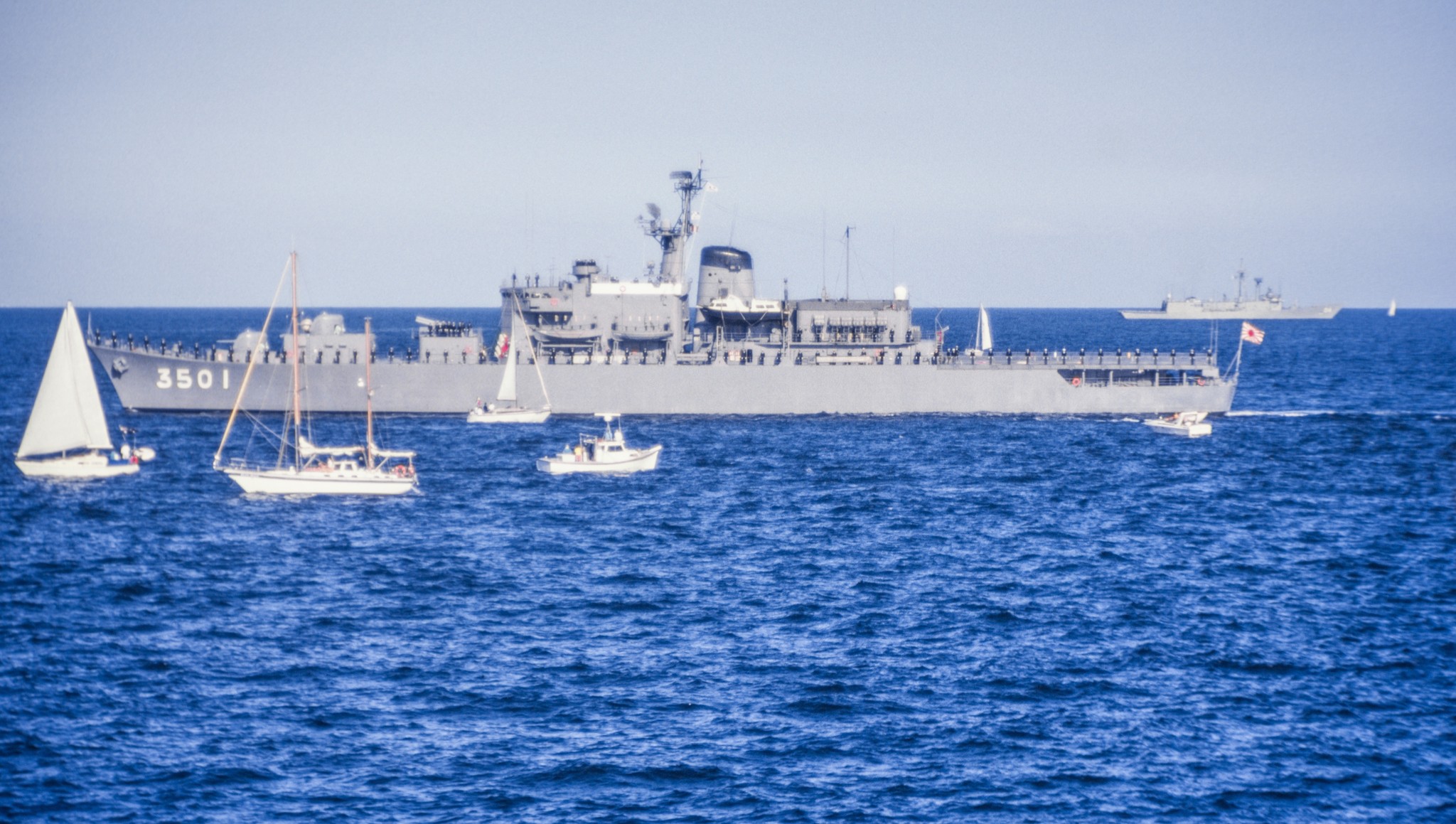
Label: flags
xmin=498 ymin=313 xmax=533 ymax=401
xmin=1241 ymin=322 xmax=1265 ymax=345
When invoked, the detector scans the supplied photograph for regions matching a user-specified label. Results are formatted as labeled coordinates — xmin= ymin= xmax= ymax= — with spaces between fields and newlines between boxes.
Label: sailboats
xmin=10 ymin=298 xmax=155 ymax=480
xmin=465 ymin=287 xmax=554 ymax=424
xmin=211 ymin=246 xmax=418 ymax=500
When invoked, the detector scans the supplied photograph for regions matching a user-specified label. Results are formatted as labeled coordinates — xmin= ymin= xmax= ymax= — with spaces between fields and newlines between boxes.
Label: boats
xmin=532 ymin=414 xmax=662 ymax=479
xmin=537 ymin=327 xmax=600 ymax=344
xmin=612 ymin=331 xmax=674 ymax=344
xmin=85 ymin=32 xmax=1243 ymax=425
xmin=1144 ymin=411 xmax=1214 ymax=439
xmin=1120 ymin=266 xmax=1344 ymax=321
xmin=699 ymin=293 xmax=790 ymax=324
